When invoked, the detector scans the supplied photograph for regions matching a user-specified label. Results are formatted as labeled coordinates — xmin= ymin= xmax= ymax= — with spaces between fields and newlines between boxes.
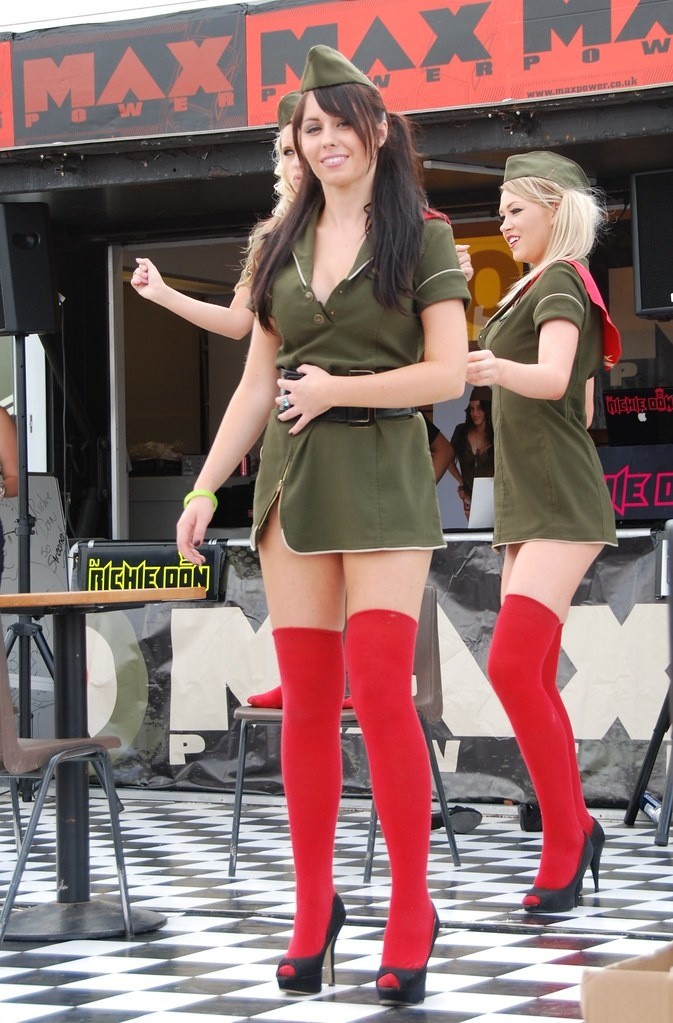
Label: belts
xmin=278 ymin=369 xmax=418 ymax=427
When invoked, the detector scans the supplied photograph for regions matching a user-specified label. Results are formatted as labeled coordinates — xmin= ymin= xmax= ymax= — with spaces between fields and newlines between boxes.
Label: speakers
xmin=0 ymin=202 xmax=59 ymax=336
xmin=630 ymin=167 xmax=673 ymax=322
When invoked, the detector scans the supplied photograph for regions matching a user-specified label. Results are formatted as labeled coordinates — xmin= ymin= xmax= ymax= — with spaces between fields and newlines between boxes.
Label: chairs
xmin=228 ymin=583 xmax=461 ymax=883
xmin=0 ymin=622 xmax=134 ymax=943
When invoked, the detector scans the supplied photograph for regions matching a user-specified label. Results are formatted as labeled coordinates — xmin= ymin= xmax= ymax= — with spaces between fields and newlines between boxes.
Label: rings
xmin=282 ymin=394 xmax=289 ymax=409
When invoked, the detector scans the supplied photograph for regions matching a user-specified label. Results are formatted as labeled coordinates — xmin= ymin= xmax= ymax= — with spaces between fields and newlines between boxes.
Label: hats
xmin=504 ymin=150 xmax=592 ymax=196
xmin=277 ymin=91 xmax=305 ymax=132
xmin=301 ymin=44 xmax=377 ymax=92
xmin=470 ymin=386 xmax=492 ymax=402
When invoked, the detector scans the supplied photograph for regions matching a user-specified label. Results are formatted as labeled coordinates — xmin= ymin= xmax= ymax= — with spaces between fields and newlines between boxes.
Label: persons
xmin=177 ymin=46 xmax=467 ymax=1006
xmin=0 ymin=405 xmax=19 ymax=585
xmin=130 ymin=90 xmax=474 ymax=709
xmin=468 ymin=151 xmax=621 ymax=913
xmin=423 ymin=386 xmax=494 ymax=521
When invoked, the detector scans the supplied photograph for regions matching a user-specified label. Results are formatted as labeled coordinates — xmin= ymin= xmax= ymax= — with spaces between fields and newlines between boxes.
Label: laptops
xmin=601 ymin=386 xmax=673 ymax=447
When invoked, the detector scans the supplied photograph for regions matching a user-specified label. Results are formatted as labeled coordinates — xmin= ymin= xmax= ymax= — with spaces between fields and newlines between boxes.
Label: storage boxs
xmin=579 ymin=939 xmax=673 ymax=1023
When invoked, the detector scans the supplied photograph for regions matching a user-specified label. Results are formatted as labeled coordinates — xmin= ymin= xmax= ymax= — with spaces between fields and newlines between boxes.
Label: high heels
xmin=276 ymin=894 xmax=347 ymax=994
xmin=377 ymin=901 xmax=441 ymax=1006
xmin=431 ymin=807 xmax=482 ymax=834
xmin=521 ymin=832 xmax=593 ymax=912
xmin=576 ymin=816 xmax=605 ymax=896
xmin=519 ymin=802 xmax=542 ymax=831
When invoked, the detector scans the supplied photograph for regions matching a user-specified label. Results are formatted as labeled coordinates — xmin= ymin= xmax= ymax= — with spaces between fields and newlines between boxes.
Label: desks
xmin=0 ymin=586 xmax=205 ymax=942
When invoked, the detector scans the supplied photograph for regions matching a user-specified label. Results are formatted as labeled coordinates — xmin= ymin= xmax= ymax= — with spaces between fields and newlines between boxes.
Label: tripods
xmin=0 ymin=333 xmax=123 ymax=814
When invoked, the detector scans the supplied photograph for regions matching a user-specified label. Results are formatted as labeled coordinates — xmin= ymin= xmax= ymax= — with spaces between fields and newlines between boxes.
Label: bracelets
xmin=183 ymin=489 xmax=218 ymax=512
